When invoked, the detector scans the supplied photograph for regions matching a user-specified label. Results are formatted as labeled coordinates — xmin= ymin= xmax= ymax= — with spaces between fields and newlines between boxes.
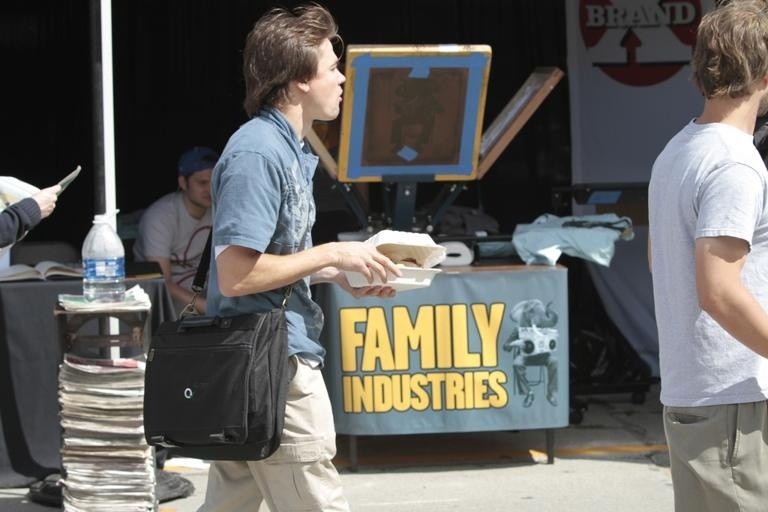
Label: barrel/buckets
xmin=80 ymin=208 xmax=124 ymax=301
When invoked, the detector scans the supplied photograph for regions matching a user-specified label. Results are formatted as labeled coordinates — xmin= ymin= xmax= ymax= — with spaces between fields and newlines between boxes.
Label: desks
xmin=1 ymin=274 xmax=198 ymax=483
xmin=316 ymin=260 xmax=572 ymax=475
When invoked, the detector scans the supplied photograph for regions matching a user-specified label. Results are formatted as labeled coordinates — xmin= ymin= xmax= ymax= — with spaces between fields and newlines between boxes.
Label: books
xmin=0 ymin=165 xmax=85 ymax=251
xmin=57 ymin=349 xmax=161 ymax=512
xmin=0 ymin=259 xmax=86 ymax=282
xmin=57 ymin=281 xmax=154 ymax=313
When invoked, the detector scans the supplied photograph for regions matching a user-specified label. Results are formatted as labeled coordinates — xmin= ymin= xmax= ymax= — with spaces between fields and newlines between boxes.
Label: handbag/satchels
xmin=144 ymin=305 xmax=288 ymax=461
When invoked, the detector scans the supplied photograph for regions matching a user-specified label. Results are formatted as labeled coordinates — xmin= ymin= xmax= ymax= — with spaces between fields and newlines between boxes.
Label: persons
xmin=0 ymin=183 xmax=62 ymax=248
xmin=191 ymin=2 xmax=401 ymax=512
xmin=646 ymin=1 xmax=768 ymax=510
xmin=135 ymin=148 xmax=221 ymax=323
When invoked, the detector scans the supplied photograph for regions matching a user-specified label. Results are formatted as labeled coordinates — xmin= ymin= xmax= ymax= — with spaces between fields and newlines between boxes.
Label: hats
xmin=177 ymin=145 xmax=218 ymax=176
